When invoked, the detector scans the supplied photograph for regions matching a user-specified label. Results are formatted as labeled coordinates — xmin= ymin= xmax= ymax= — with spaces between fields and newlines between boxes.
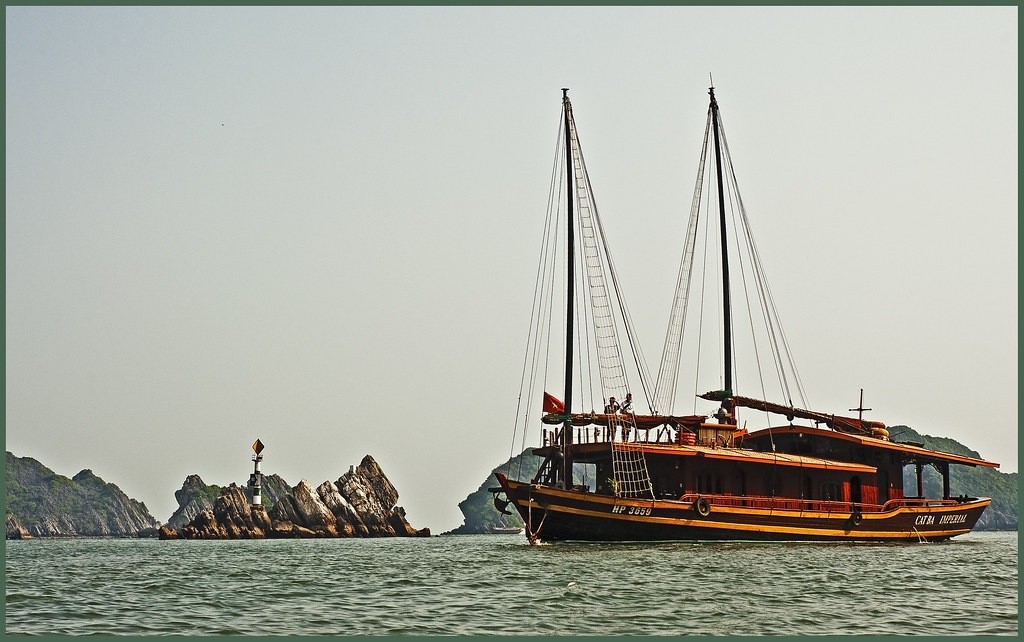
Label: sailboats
xmin=487 ymin=71 xmax=1000 ymax=546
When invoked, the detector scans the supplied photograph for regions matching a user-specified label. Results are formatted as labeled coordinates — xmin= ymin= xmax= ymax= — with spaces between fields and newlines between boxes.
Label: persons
xmin=604 ymin=397 xmax=621 ymax=443
xmin=619 ymin=393 xmax=635 ymax=442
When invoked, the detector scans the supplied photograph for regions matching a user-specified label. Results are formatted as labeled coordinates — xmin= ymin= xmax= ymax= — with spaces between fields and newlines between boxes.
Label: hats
xmin=626 ymin=393 xmax=631 ymax=398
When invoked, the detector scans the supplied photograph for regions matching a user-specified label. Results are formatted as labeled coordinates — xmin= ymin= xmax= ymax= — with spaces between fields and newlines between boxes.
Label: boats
xmin=490 ymin=525 xmax=523 ymax=534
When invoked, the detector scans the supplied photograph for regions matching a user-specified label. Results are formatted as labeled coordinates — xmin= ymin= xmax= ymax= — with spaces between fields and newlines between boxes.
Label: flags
xmin=542 ymin=391 xmax=565 ymax=413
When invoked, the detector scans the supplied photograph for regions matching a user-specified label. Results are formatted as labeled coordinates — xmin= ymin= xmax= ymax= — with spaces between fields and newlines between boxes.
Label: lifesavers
xmin=868 ymin=427 xmax=890 ymax=443
xmin=694 ymin=498 xmax=711 ymax=516
xmin=674 ymin=433 xmax=697 ymax=445
xmin=850 ymin=511 xmax=863 ymax=526
